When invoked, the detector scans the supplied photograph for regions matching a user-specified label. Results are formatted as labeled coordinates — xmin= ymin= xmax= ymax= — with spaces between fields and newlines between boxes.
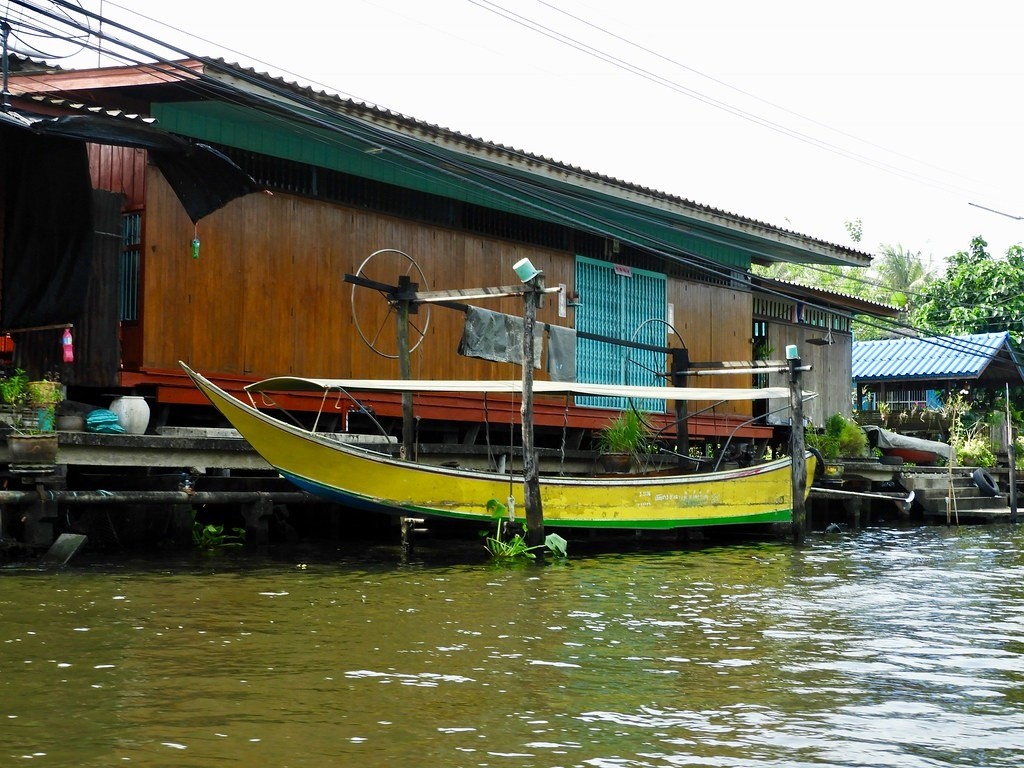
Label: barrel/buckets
xmin=512 ymin=258 xmax=539 ymax=282
xmin=28 ymin=382 xmax=58 ymax=402
xmin=785 ymin=345 xmax=799 ymax=359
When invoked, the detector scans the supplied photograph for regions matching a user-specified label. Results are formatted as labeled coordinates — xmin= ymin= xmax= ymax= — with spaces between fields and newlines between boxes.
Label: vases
xmin=27 ymin=379 xmax=61 ymax=408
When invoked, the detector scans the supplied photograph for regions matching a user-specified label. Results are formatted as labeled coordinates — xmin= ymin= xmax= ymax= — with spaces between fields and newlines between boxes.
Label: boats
xmin=176 ymin=247 xmax=826 ymax=547
xmin=860 ymin=424 xmax=958 ymax=467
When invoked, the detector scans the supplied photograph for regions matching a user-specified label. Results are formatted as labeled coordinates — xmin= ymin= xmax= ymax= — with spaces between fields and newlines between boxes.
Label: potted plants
xmin=593 ymin=408 xmax=650 ymax=472
xmin=961 ymin=428 xmax=995 ymax=466
xmin=816 ymin=415 xmax=847 ymax=479
xmin=0 ymin=367 xmax=59 ymax=465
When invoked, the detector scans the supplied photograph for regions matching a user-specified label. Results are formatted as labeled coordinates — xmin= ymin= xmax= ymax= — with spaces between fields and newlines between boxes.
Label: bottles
xmin=62 ymin=329 xmax=74 ymax=362
